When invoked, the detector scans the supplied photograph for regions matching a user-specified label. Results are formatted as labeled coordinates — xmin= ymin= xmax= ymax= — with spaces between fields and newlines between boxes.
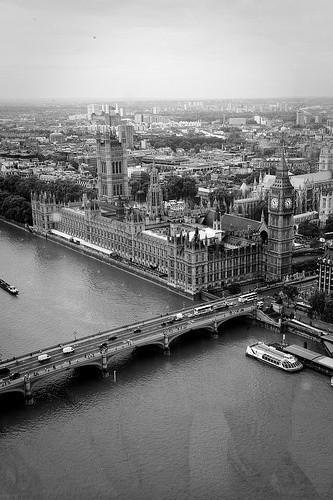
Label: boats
xmin=246 ymin=341 xmax=333 ymax=388
xmin=0 ymin=279 xmax=19 ymax=295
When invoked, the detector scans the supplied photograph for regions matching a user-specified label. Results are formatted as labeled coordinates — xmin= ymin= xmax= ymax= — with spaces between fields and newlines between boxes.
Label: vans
xmin=257 ymin=301 xmax=264 ymax=306
xmin=0 ymin=367 xmax=10 ymax=376
xmin=38 ymin=346 xmax=75 ymax=362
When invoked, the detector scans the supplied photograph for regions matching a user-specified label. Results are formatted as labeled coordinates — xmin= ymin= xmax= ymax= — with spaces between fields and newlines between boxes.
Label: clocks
xmin=270 ymin=197 xmax=279 ymax=210
xmin=284 ymin=198 xmax=293 ymax=210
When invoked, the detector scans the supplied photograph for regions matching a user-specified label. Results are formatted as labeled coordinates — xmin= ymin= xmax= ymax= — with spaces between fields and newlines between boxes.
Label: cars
xmin=134 ymin=329 xmax=141 ymax=333
xmin=10 ymin=372 xmax=20 ymax=379
xmin=161 ymin=313 xmax=195 ymax=326
xmin=108 ymin=336 xmax=117 ymax=341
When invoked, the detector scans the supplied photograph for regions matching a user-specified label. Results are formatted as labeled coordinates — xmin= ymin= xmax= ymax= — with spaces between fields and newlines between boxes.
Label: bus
xmin=296 ymin=301 xmax=312 ymax=312
xmin=238 ymin=293 xmax=258 ymax=302
xmin=194 ymin=304 xmax=214 ymax=317
